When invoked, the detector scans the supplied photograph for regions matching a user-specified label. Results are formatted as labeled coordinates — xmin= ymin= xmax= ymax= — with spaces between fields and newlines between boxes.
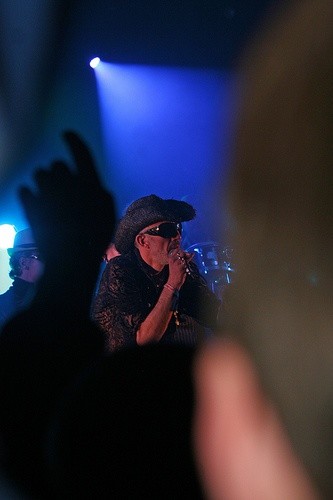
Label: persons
xmin=91 ymin=194 xmax=218 ymax=354
xmin=193 ymin=2 xmax=333 ymax=500
xmin=0 ymin=229 xmax=44 ymax=331
xmin=0 ymin=131 xmax=198 ymax=500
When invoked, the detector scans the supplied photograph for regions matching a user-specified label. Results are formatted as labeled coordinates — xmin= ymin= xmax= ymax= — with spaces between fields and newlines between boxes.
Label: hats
xmin=6 ymin=228 xmax=38 ymax=257
xmin=114 ymin=194 xmax=196 ymax=255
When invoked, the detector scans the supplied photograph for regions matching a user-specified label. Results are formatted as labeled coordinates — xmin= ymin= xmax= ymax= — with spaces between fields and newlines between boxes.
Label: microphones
xmin=169 ymin=249 xmax=207 ymax=285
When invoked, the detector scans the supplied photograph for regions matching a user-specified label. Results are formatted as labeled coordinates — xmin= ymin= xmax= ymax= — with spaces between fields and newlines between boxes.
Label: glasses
xmin=139 ymin=222 xmax=184 ymax=238
xmin=30 ymin=255 xmax=46 ymax=264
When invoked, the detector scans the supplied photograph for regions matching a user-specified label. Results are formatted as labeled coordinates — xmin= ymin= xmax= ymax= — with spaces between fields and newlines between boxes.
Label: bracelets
xmin=163 ymin=283 xmax=180 ymax=298
